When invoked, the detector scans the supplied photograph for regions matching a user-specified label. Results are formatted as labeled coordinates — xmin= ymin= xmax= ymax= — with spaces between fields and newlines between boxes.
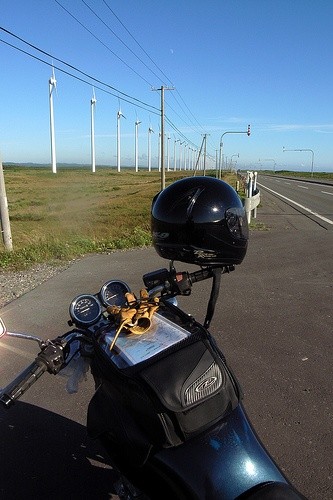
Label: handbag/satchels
xmin=91 ymin=297 xmax=244 ymax=450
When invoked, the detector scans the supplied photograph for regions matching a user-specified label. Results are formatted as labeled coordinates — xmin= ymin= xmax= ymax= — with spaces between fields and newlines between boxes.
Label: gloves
xmin=107 ymin=289 xmax=159 ymax=335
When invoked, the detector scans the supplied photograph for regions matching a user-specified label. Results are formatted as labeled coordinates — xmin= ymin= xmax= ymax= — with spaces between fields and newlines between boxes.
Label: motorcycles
xmin=1 ymin=259 xmax=312 ymax=499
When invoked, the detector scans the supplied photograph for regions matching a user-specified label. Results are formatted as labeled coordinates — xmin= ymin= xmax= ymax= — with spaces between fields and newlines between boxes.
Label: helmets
xmin=150 ymin=177 xmax=248 ymax=266
